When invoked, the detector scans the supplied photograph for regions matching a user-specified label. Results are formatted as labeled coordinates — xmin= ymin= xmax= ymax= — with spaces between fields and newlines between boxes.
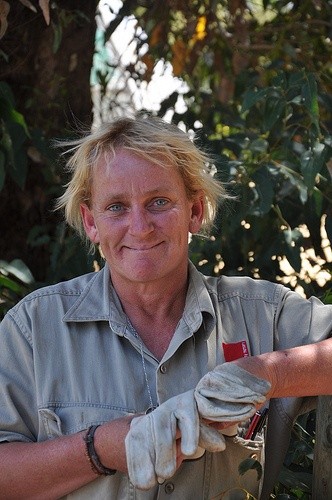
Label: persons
xmin=1 ymin=114 xmax=332 ymax=500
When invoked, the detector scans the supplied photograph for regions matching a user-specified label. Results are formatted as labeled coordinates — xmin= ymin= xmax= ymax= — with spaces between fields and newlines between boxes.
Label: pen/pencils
xmin=244 ymin=413 xmax=260 ymax=444
xmin=251 ymin=407 xmax=268 ymax=441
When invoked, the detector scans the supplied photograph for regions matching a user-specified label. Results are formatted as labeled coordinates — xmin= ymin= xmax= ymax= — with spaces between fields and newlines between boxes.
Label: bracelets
xmin=84 ymin=425 xmax=117 ymax=477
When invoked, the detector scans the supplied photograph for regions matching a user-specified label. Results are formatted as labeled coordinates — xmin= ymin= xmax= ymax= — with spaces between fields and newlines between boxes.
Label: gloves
xmin=194 ymin=353 xmax=270 ymax=437
xmin=125 ymin=389 xmax=225 ymax=492
